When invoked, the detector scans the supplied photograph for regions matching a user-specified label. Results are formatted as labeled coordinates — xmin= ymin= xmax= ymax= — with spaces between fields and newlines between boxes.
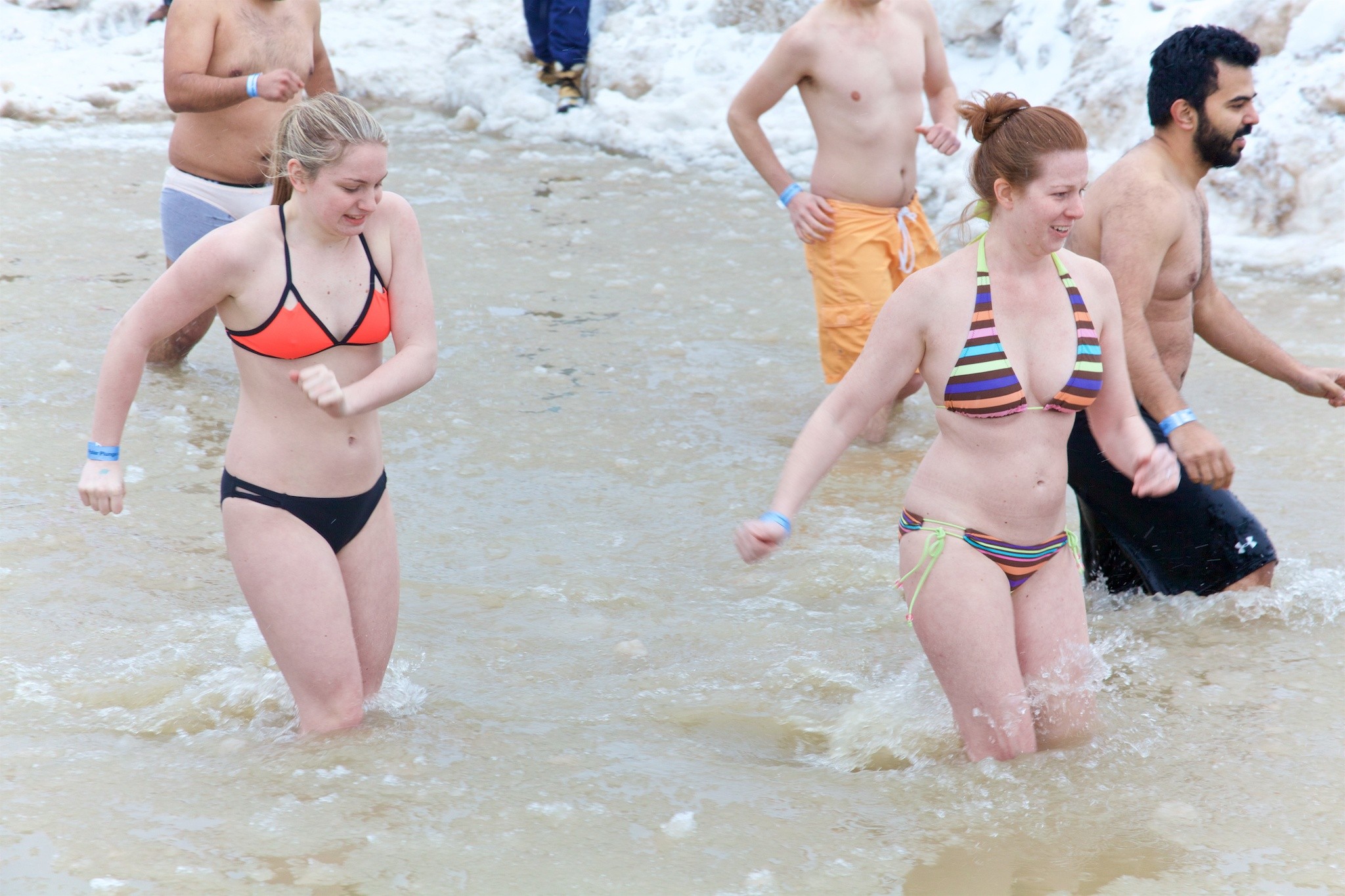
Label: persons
xmin=1062 ymin=24 xmax=1345 ymax=597
xmin=146 ymin=0 xmax=339 ymax=367
xmin=523 ymin=0 xmax=590 ymax=113
xmin=726 ymin=0 xmax=961 ymax=446
xmin=77 ymin=93 xmax=437 ymax=733
xmin=735 ymin=92 xmax=1181 ymax=764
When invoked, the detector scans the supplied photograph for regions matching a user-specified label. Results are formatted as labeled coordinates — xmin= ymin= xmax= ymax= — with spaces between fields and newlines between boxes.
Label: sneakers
xmin=529 ymin=51 xmax=556 ymax=86
xmin=553 ymin=62 xmax=585 ymax=112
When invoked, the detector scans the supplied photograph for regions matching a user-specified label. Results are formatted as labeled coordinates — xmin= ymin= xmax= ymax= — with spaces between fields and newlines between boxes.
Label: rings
xmin=1166 ymin=468 xmax=1171 ymax=479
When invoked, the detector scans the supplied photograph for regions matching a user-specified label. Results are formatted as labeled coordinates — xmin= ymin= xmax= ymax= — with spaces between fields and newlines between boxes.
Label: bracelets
xmin=87 ymin=441 xmax=119 ymax=461
xmin=246 ymin=73 xmax=259 ymax=97
xmin=776 ymin=183 xmax=802 ymax=210
xmin=1158 ymin=408 xmax=1196 ymax=437
xmin=759 ymin=511 xmax=791 ymax=532
